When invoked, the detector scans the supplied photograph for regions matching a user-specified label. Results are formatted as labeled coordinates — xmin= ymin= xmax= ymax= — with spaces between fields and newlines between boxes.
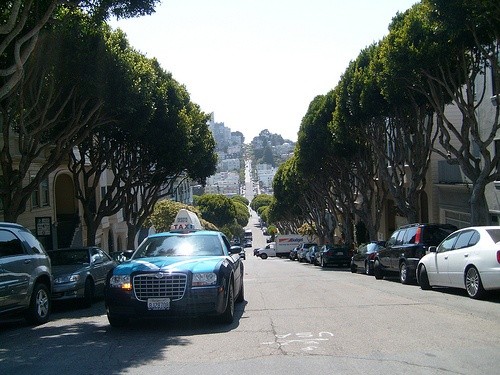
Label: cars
xmin=107 ymin=249 xmax=138 ymax=267
xmin=350 ymin=240 xmax=387 ymax=275
xmin=104 ymin=209 xmax=244 ymax=328
xmin=415 ymin=226 xmax=500 ymax=298
xmin=231 ymin=245 xmax=245 ymax=259
xmin=258 ymin=217 xmax=275 ymax=243
xmin=288 ymin=242 xmax=351 ymax=269
xmin=44 ymin=247 xmax=123 ymax=307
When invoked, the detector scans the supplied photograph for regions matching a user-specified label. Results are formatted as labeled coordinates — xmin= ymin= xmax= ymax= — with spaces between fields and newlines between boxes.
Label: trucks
xmin=254 ymin=235 xmax=309 ymax=259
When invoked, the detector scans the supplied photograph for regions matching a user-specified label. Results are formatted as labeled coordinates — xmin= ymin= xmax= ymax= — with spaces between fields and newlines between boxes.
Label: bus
xmin=241 ymin=230 xmax=253 ymax=247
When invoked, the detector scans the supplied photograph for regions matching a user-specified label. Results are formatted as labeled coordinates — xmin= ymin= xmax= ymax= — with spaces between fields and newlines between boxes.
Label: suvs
xmin=370 ymin=222 xmax=460 ymax=285
xmin=0 ymin=222 xmax=54 ymax=326
